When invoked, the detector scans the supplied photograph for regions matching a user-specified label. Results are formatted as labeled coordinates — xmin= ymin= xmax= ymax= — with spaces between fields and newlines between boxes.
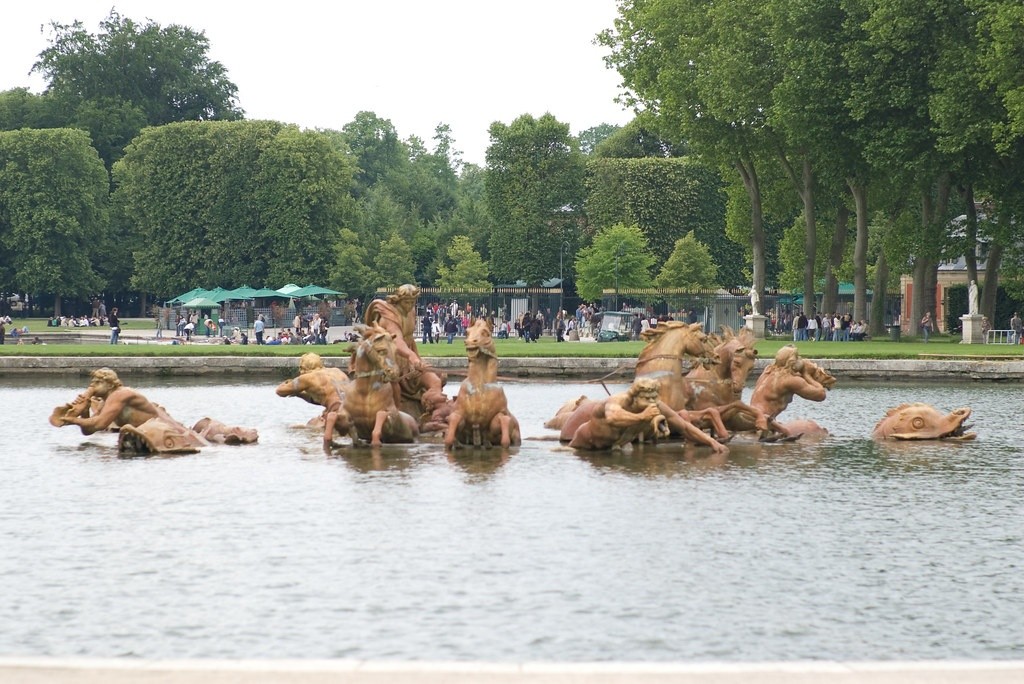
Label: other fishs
xmin=117 ymin=421 xmax=205 ymax=452
xmin=870 ymin=401 xmax=977 ymax=441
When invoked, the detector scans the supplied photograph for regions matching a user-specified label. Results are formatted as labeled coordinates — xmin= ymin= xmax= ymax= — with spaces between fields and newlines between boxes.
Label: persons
xmin=750 ymin=345 xmax=826 ymax=421
xmin=275 ymin=353 xmax=352 ymax=425
xmin=155 ymin=318 xmax=162 ymax=340
xmin=922 ymin=311 xmax=934 ymax=344
xmin=568 ymin=376 xmax=730 ymax=454
xmin=737 ymin=300 xmax=870 ymax=341
xmin=175 ymin=310 xmax=199 ymax=340
xmin=968 ymin=280 xmax=979 ymax=315
xmin=230 ymin=327 xmax=247 ymax=345
xmin=218 ymin=296 xmax=364 ymax=323
xmin=217 ymin=316 xmax=225 ymax=337
xmin=59 ymin=367 xmax=160 ymax=435
xmin=419 ymin=299 xmax=698 ymax=344
xmin=364 ymin=284 xmax=441 ymax=410
xmin=47 ymin=297 xmax=121 ymax=345
xmin=253 ymin=311 xmax=329 ymax=345
xmin=0 ymin=314 xmax=46 ymax=346
xmin=981 ymin=317 xmax=991 ymax=344
xmin=204 ymin=312 xmax=216 ymax=337
xmin=1011 ymin=312 xmax=1024 ymax=344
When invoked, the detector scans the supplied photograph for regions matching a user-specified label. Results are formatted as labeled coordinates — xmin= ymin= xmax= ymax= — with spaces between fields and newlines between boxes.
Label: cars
xmin=0 ymin=293 xmax=20 ymax=306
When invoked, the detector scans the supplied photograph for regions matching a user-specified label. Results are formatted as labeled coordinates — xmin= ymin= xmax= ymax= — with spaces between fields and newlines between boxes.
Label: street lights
xmin=615 ymin=242 xmax=625 ymax=312
xmin=560 ymin=241 xmax=570 ymax=320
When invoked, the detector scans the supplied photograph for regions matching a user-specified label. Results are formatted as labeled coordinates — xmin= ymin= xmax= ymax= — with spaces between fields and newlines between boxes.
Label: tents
xmin=166 ymin=283 xmax=348 ymax=318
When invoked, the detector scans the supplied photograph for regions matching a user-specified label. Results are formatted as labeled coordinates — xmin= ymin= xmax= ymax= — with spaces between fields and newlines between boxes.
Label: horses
xmin=549 ymin=320 xmax=789 ymax=453
xmin=322 ymin=322 xmax=522 ymax=451
